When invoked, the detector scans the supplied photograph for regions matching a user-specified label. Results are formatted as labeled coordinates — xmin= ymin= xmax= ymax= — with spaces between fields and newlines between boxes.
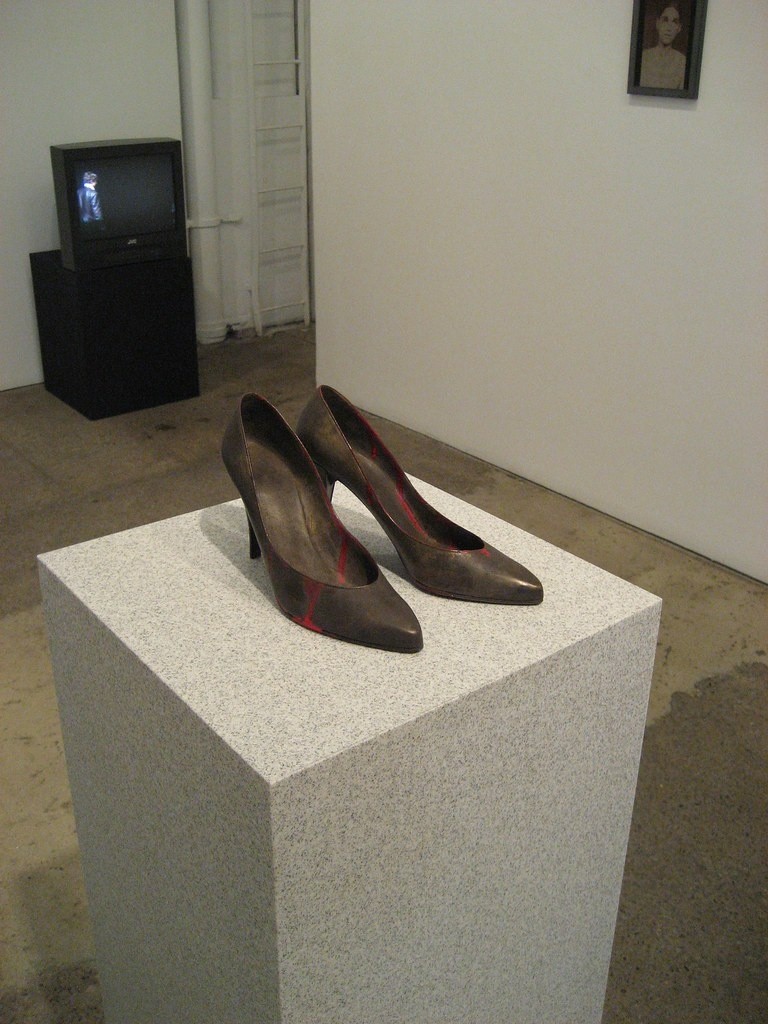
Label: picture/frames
xmin=627 ymin=0 xmax=709 ymax=100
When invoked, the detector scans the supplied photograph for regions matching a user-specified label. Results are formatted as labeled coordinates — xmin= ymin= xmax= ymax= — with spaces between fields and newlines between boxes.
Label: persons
xmin=640 ymin=0 xmax=686 ymax=90
xmin=76 ymin=170 xmax=104 ymax=222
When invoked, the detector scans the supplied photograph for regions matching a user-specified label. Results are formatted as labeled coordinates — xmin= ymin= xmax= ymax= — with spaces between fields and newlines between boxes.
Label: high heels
xmin=222 ymin=391 xmax=424 ymax=653
xmin=297 ymin=385 xmax=545 ymax=605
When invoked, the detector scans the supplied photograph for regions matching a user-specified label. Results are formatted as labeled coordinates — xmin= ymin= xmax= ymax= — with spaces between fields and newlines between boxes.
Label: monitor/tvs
xmin=49 ymin=136 xmax=186 ymax=271
